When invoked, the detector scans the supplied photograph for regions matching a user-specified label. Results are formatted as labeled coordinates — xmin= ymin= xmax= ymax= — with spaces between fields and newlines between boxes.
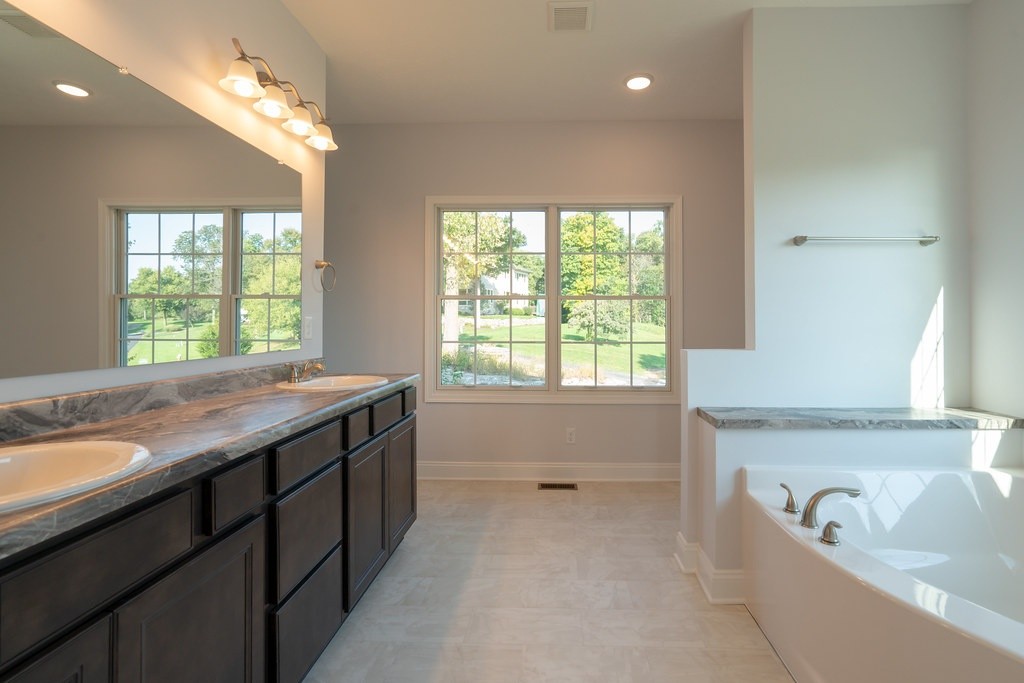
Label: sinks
xmin=0 ymin=441 xmax=152 ymax=513
xmin=276 ymin=376 xmax=388 ymax=391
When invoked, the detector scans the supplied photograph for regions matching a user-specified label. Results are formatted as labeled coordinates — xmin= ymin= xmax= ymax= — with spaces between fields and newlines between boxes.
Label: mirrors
xmin=0 ymin=0 xmax=304 ymax=380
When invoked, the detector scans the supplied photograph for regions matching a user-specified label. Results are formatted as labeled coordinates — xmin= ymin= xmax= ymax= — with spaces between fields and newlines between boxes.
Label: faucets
xmin=799 ymin=488 xmax=861 ymax=529
xmin=301 ymin=361 xmax=326 ymax=381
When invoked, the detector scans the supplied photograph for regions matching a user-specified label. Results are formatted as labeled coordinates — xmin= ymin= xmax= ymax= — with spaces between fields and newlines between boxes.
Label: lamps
xmin=217 ymin=36 xmax=338 ymax=151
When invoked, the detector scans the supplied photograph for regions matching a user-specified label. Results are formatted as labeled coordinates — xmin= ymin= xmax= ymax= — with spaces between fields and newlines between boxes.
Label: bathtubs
xmin=741 ymin=465 xmax=1024 ymax=683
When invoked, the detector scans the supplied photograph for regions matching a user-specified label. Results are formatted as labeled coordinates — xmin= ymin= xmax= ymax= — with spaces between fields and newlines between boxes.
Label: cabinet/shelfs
xmin=0 ymin=448 xmax=267 ymax=683
xmin=341 ymin=385 xmax=418 ymax=622
xmin=267 ymin=415 xmax=343 ymax=683
